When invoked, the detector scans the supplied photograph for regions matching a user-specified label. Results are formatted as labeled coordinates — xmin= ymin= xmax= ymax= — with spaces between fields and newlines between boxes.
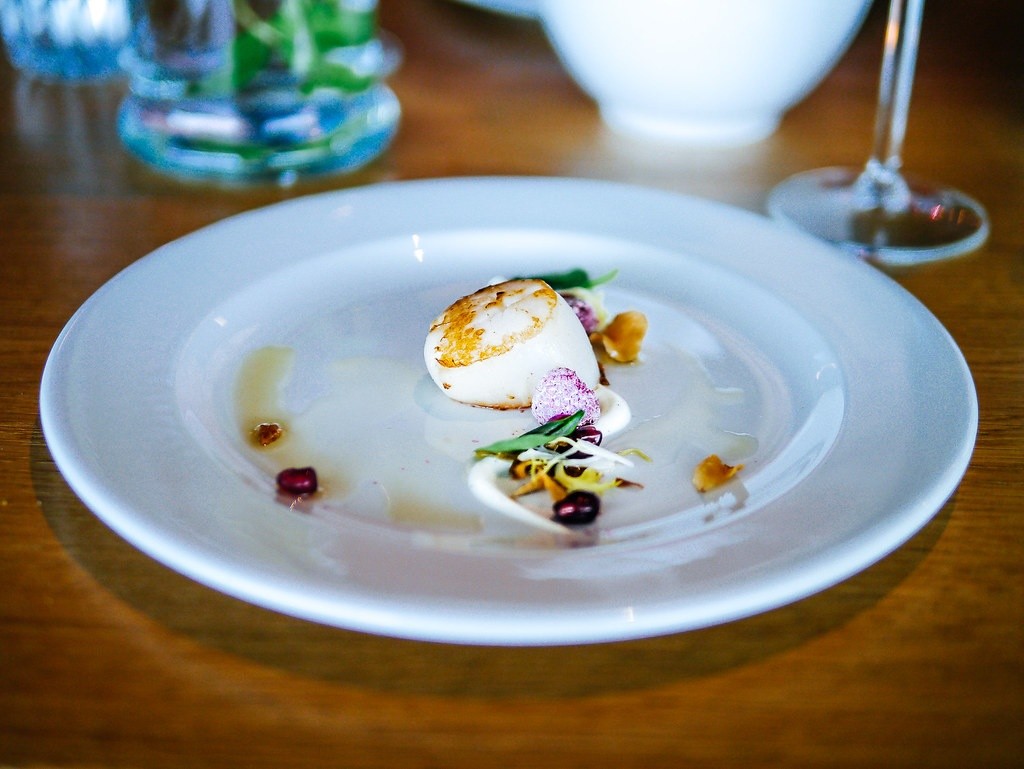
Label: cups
xmin=0 ymin=0 xmax=132 ymax=84
xmin=112 ymin=0 xmax=406 ymax=183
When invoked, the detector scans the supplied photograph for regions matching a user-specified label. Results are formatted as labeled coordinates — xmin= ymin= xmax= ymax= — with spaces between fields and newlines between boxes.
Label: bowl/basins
xmin=530 ymin=0 xmax=878 ymax=152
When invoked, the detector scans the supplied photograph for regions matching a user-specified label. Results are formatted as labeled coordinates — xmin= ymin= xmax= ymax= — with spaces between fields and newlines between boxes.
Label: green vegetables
xmin=516 ymin=266 xmax=617 ymax=290
xmin=473 ymin=409 xmax=586 ymax=452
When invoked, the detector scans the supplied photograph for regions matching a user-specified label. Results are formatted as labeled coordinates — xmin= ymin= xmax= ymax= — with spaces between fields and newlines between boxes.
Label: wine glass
xmin=766 ymin=0 xmax=990 ymax=267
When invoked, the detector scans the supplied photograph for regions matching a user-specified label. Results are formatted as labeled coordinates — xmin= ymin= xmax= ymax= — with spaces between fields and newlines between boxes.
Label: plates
xmin=39 ymin=174 xmax=980 ymax=646
